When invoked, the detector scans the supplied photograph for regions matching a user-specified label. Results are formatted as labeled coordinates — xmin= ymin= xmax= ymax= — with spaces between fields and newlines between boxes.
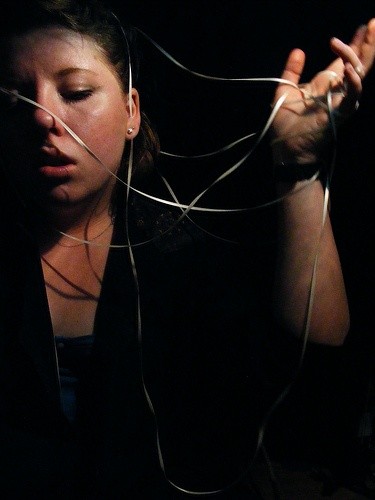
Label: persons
xmin=2 ymin=0 xmax=375 ymax=500
